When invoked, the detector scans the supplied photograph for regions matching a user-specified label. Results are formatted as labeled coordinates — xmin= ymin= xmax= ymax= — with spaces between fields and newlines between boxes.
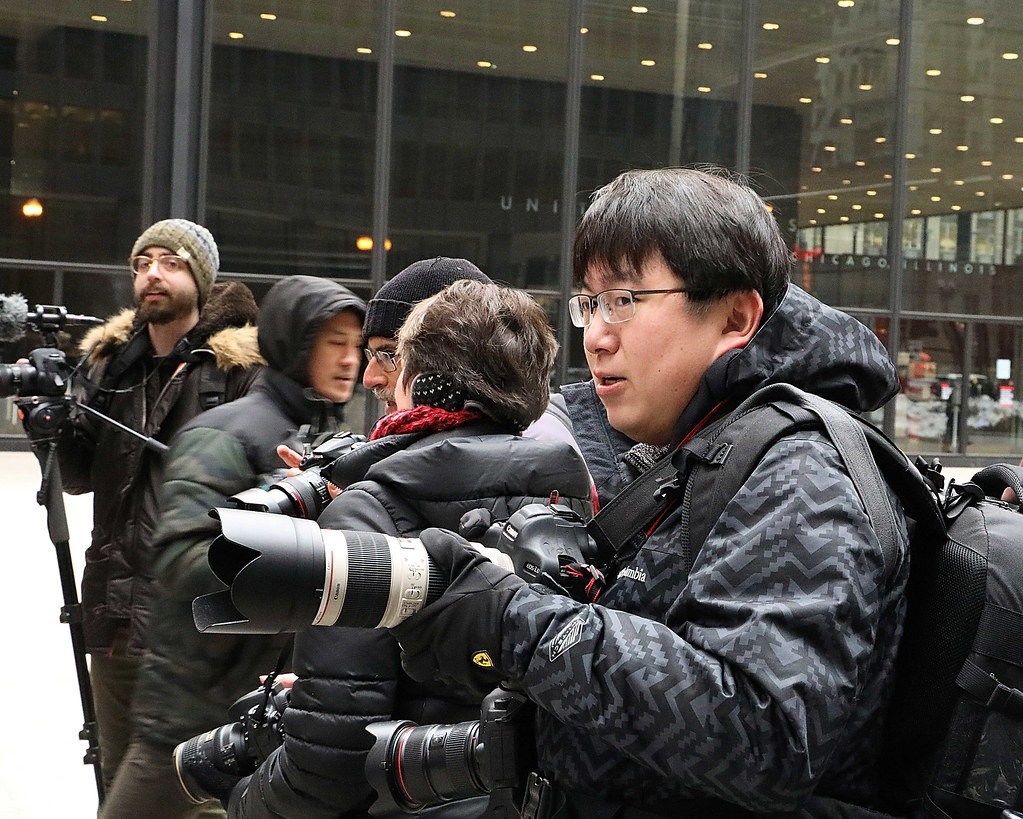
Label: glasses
xmin=126 ymin=253 xmax=188 ymax=274
xmin=566 ymin=283 xmax=688 ymax=327
xmin=363 ymin=347 xmax=400 ymax=373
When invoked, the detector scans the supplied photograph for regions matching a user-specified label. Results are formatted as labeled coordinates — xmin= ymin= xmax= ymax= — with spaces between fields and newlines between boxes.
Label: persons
xmin=96 ymin=274 xmax=370 ymax=819
xmin=388 ymin=160 xmax=917 ymax=819
xmin=186 ymin=278 xmax=594 ymax=819
xmin=15 ymin=219 xmax=270 ymax=801
xmin=276 ymin=255 xmax=600 ymax=522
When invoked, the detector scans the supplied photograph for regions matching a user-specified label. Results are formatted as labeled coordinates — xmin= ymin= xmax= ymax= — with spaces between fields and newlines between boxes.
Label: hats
xmin=129 ymin=218 xmax=221 ymax=311
xmin=362 ymin=256 xmax=497 ymax=344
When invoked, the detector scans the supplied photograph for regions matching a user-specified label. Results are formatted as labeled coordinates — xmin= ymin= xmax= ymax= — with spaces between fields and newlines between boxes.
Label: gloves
xmin=387 ymin=524 xmax=528 ymax=701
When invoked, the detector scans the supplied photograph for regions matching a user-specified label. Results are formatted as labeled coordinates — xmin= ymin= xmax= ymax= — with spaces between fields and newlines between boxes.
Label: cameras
xmin=1 ymin=295 xmax=107 ymax=438
xmin=187 ymin=503 xmax=611 ymax=636
xmin=172 ymin=675 xmax=293 ymax=810
xmin=227 ymin=429 xmax=372 ymax=521
xmin=361 ymin=669 xmax=570 ymax=818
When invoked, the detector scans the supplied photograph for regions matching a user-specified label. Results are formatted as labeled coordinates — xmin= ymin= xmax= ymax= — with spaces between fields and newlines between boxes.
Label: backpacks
xmin=681 ymin=382 xmax=1023 ymax=817
